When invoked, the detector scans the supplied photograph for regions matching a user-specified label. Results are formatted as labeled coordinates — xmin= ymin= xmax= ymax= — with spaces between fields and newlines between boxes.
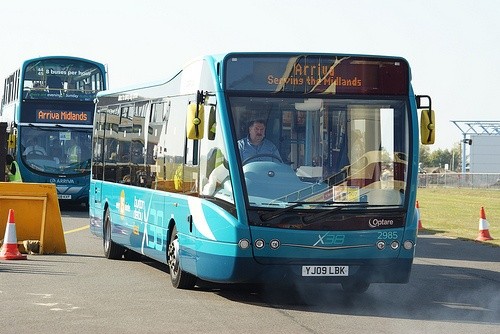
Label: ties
xmin=33 ymin=146 xmax=36 ymax=156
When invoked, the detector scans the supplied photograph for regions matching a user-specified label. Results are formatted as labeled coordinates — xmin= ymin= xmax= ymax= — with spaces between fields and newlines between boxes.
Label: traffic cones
xmin=0 ymin=208 xmax=27 ymax=261
xmin=415 ymin=200 xmax=422 ymax=231
xmin=477 ymin=206 xmax=493 ymax=240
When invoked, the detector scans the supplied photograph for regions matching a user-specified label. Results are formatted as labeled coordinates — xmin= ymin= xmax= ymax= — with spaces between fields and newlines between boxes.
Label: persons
xmin=22 ymin=138 xmax=46 ymax=155
xmin=67 ymin=137 xmax=81 ymax=162
xmin=53 ymin=139 xmax=60 ymax=146
xmin=47 ymin=68 xmax=63 ymax=94
xmin=33 ymin=84 xmax=46 ymax=94
xmin=237 ymin=119 xmax=284 ymax=163
xmin=85 ymin=84 xmax=91 ymax=94
xmin=418 ymin=163 xmax=425 ymax=174
xmin=5 ymin=154 xmax=22 ymax=182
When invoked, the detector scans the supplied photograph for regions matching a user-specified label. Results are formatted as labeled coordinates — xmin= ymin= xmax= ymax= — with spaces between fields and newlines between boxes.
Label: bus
xmin=87 ymin=51 xmax=436 ymax=298
xmin=0 ymin=55 xmax=109 ymax=212
